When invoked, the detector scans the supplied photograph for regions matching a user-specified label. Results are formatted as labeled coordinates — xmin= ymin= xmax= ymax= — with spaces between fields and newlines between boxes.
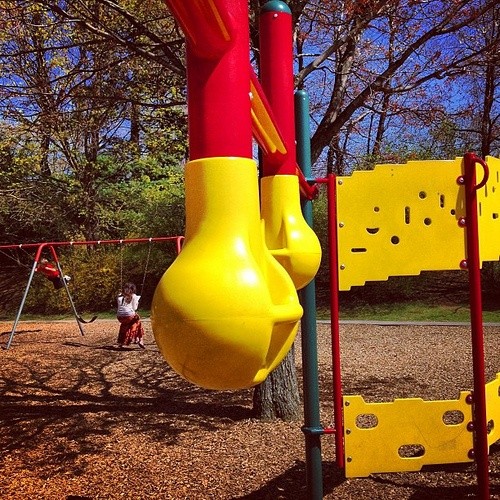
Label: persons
xmin=116 ymin=282 xmax=147 ymax=349
xmin=33 ymin=256 xmax=72 ymax=290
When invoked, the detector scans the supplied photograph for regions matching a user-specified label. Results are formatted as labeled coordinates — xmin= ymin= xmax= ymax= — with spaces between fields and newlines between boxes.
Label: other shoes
xmin=139 ymin=341 xmax=145 ymax=349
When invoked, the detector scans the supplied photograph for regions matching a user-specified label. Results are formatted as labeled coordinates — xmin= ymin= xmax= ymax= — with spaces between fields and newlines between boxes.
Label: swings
xmin=68 ymin=246 xmax=103 ymax=324
xmin=118 ymin=242 xmax=152 ymax=328
xmin=0 ymin=246 xmax=62 ymax=284
